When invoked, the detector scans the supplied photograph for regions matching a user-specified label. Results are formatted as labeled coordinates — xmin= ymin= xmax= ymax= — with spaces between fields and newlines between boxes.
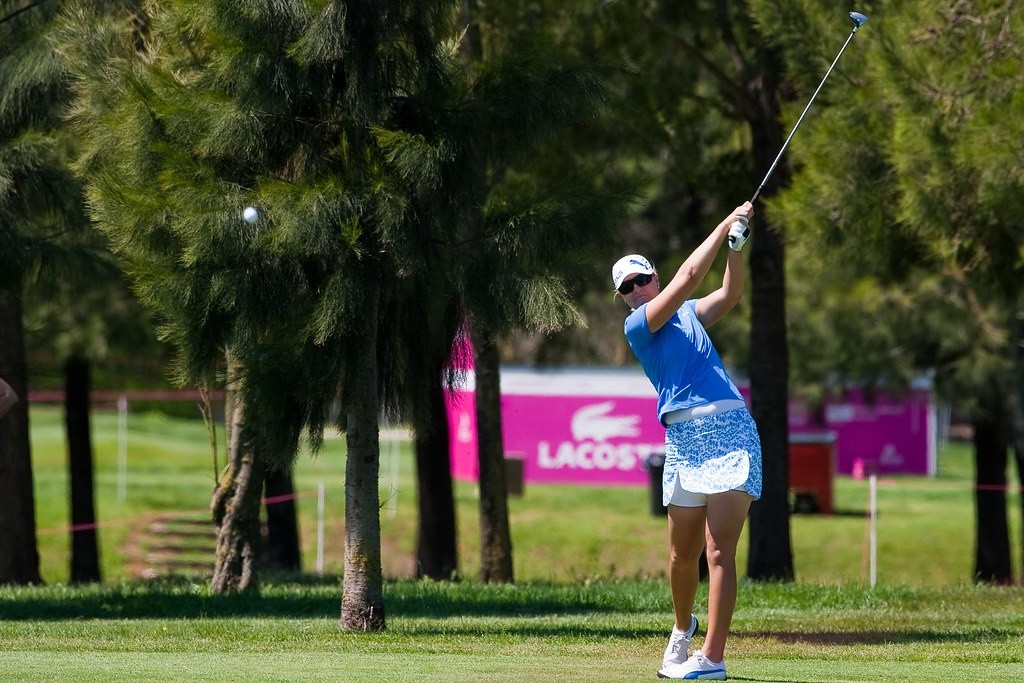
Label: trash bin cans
xmin=648 ymin=453 xmax=667 ymax=515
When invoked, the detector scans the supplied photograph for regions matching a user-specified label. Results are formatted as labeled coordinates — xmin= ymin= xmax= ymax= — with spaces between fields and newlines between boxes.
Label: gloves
xmin=728 ymin=215 xmax=751 ymax=252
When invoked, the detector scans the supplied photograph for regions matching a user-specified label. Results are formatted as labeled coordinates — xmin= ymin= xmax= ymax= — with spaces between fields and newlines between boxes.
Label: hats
xmin=612 ymin=254 xmax=654 ymax=290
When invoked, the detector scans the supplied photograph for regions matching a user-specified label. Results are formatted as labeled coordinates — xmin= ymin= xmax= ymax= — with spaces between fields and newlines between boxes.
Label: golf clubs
xmin=728 ymin=11 xmax=869 ymax=243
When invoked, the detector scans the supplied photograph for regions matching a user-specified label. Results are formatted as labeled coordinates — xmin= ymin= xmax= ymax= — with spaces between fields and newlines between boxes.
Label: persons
xmin=612 ymin=202 xmax=762 ymax=681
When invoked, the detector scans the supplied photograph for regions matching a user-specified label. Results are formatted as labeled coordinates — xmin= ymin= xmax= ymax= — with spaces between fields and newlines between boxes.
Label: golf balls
xmin=244 ymin=206 xmax=259 ymax=225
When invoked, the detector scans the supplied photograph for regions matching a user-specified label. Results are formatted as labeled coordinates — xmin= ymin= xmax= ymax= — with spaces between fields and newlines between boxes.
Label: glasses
xmin=618 ymin=273 xmax=653 ymax=295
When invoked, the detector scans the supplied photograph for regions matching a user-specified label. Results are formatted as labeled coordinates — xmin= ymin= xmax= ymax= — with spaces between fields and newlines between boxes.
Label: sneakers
xmin=663 ymin=613 xmax=700 ymax=669
xmin=656 ymin=650 xmax=726 ymax=681
